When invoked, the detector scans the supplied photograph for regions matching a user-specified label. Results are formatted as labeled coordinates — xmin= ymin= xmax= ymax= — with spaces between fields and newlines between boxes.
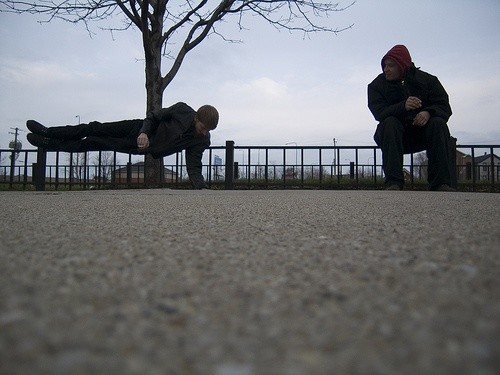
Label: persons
xmin=367 ymin=45 xmax=456 ymax=192
xmin=26 ymin=102 xmax=219 ymax=191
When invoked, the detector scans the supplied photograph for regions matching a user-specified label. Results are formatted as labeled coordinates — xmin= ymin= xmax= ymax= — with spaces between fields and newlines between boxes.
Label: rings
xmin=422 ymin=121 xmax=423 ymax=123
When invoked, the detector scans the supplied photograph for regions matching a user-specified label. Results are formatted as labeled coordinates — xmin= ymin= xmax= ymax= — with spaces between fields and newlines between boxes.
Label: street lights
xmin=76 ymin=115 xmax=80 ymax=165
xmin=333 ymin=138 xmax=338 ymax=173
xmin=286 ymin=142 xmax=298 ymax=171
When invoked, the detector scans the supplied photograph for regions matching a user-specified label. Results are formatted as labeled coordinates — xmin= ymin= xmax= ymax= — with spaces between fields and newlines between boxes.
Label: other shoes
xmin=386 ymin=184 xmax=399 ymax=190
xmin=430 ymin=184 xmax=456 ymax=192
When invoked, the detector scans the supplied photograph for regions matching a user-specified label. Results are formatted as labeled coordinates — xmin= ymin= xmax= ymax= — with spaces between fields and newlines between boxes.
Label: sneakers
xmin=26 ymin=120 xmax=48 ymax=137
xmin=26 ymin=133 xmax=49 ymax=149
